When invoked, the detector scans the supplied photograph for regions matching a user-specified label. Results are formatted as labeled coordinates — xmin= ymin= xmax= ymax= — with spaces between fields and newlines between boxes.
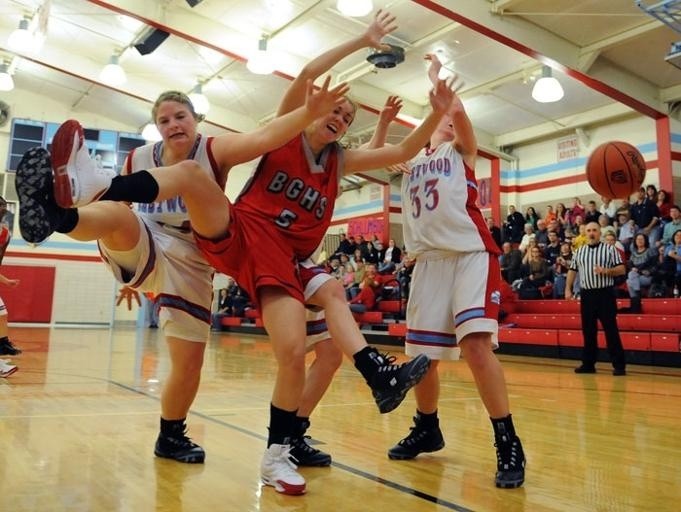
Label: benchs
xmin=213 ymin=301 xmax=265 ymax=329
xmin=499 ymin=292 xmax=681 ymax=354
xmin=334 ymin=262 xmax=406 ymax=336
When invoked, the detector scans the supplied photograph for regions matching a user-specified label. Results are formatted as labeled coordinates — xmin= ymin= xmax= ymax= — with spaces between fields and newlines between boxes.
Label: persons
xmin=322 ymin=233 xmax=414 ymax=312
xmin=355 ymin=54 xmax=525 ymax=488
xmin=486 ymin=184 xmax=681 ymax=315
xmin=211 ymin=276 xmax=249 ymax=332
xmin=0 ymin=197 xmax=22 ymax=356
xmin=280 ymin=93 xmax=431 ymax=465
xmin=0 ymin=99 xmax=19 ymax=379
xmin=15 ymin=72 xmax=352 ymax=466
xmin=51 ymin=8 xmax=466 ymax=495
xmin=565 ymin=220 xmax=626 ymax=377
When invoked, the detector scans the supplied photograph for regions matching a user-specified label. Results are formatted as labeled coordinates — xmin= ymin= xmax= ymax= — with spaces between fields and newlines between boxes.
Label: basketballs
xmin=587 ymin=143 xmax=647 ymax=198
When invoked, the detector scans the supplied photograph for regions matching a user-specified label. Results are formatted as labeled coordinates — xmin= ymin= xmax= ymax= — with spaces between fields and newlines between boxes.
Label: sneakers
xmin=51 ymin=119 xmax=112 ymax=209
xmin=1 ymin=336 xmax=21 ymax=377
xmin=367 ymin=348 xmax=430 ymax=414
xmin=389 ymin=416 xmax=444 ymax=459
xmin=494 ymin=434 xmax=525 ymax=487
xmin=155 ymin=424 xmax=205 ymax=463
xmin=260 ymin=421 xmax=331 ymax=495
xmin=15 ymin=148 xmax=52 ymax=243
xmin=575 ymin=365 xmax=595 ymax=373
xmin=613 ymin=369 xmax=625 ymax=375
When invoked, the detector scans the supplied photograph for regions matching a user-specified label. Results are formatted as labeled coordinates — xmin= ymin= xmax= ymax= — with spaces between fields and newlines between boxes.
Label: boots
xmin=619 ymin=292 xmax=641 ymax=313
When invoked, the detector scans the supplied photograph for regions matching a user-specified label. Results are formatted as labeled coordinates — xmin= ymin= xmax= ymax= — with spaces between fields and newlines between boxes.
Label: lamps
xmin=5 ymin=19 xmax=34 ymax=48
xmin=530 ymin=65 xmax=565 ymax=103
xmin=246 ymin=33 xmax=276 ymax=74
xmin=0 ymin=63 xmax=14 ymax=91
xmin=100 ymin=52 xmax=127 ymax=84
xmin=185 ymin=83 xmax=210 ymax=113
